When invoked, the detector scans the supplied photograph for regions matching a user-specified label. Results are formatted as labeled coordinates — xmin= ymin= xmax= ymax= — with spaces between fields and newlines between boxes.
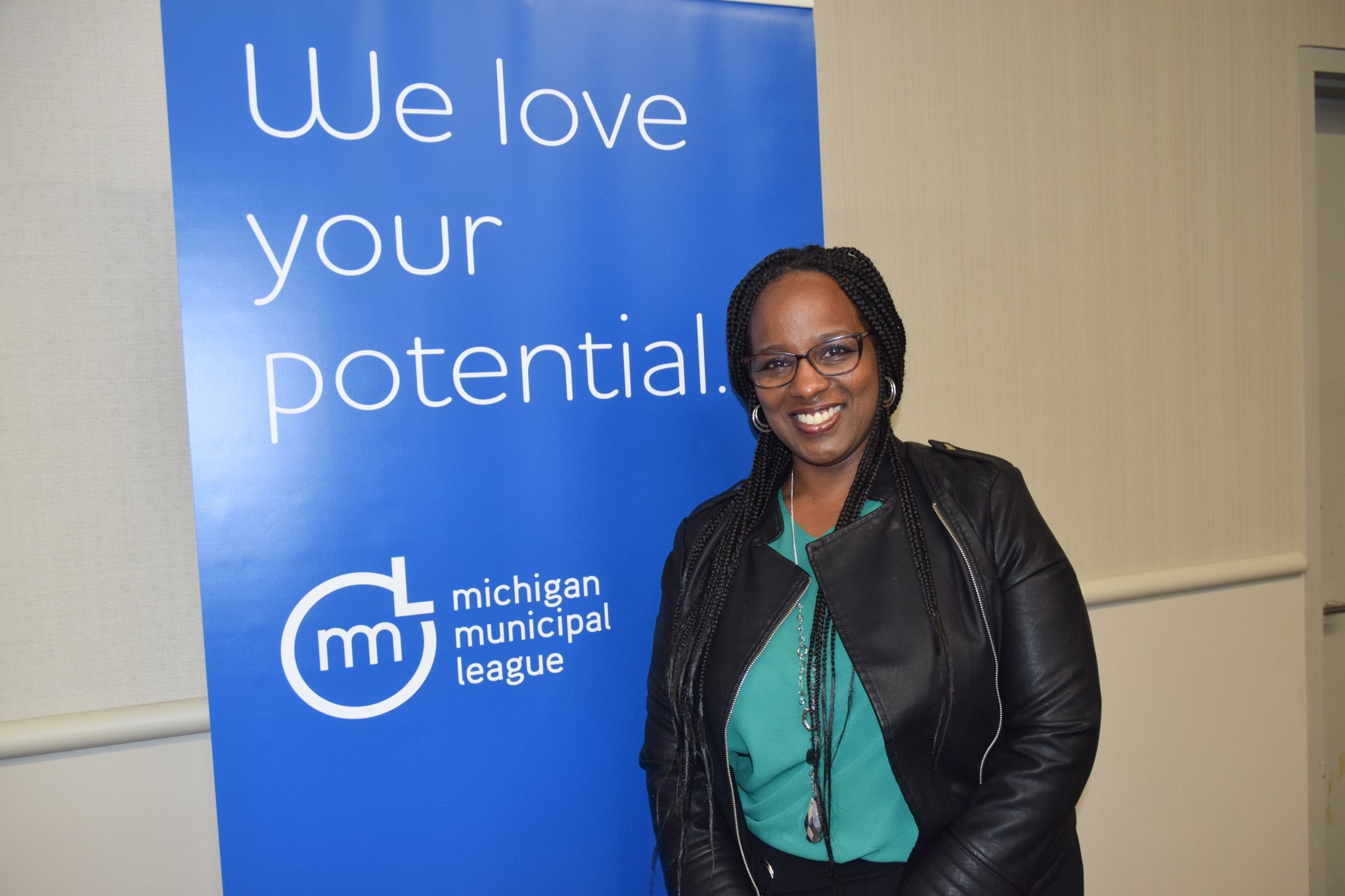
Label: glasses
xmin=744 ymin=327 xmax=872 ymax=389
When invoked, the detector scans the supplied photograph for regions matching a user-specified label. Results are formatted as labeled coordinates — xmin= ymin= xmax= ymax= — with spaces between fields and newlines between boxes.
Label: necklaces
xmin=788 ymin=460 xmax=825 ymax=845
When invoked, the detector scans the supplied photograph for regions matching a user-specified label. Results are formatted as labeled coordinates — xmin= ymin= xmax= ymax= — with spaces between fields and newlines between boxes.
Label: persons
xmin=635 ymin=243 xmax=1102 ymax=896
